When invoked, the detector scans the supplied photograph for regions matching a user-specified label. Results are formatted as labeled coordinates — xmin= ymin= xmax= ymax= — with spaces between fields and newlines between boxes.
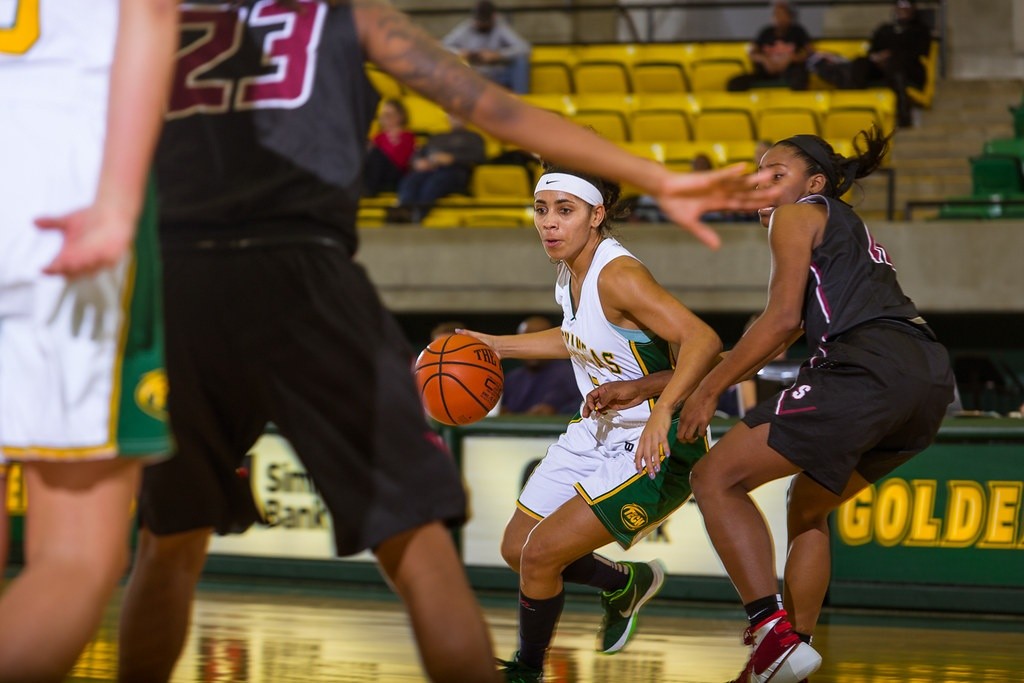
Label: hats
xmin=473 ymin=3 xmax=493 ymax=19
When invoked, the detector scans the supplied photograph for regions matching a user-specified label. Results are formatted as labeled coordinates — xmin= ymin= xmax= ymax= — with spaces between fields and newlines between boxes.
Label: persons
xmin=0 ymin=0 xmax=181 ymax=683
xmin=813 ymin=1 xmax=933 ymax=128
xmin=581 ymin=119 xmax=959 ymax=683
xmin=118 ymin=0 xmax=791 ymax=683
xmin=726 ymin=0 xmax=819 ymax=92
xmin=454 ymin=164 xmax=723 ymax=683
xmin=366 ymin=95 xmax=490 ymax=224
xmin=442 ymin=0 xmax=533 ymax=95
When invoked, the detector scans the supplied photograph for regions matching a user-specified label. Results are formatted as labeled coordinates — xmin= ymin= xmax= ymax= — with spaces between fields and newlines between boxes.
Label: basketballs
xmin=414 ymin=333 xmax=504 ymax=426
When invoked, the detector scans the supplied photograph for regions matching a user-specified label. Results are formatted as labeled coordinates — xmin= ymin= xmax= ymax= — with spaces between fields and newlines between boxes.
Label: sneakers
xmin=725 ymin=610 xmax=822 ymax=683
xmin=593 ymin=561 xmax=664 ymax=655
xmin=492 ymin=649 xmax=544 ymax=683
xmin=793 ymin=629 xmax=814 ymax=683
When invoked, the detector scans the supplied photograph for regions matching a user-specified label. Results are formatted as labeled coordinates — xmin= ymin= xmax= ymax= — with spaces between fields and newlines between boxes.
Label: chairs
xmin=363 ymin=38 xmax=1024 ymax=225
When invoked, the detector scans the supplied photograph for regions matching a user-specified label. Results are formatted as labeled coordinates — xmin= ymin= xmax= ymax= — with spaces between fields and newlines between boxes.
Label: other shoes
xmin=384 ymin=205 xmax=422 ymax=223
